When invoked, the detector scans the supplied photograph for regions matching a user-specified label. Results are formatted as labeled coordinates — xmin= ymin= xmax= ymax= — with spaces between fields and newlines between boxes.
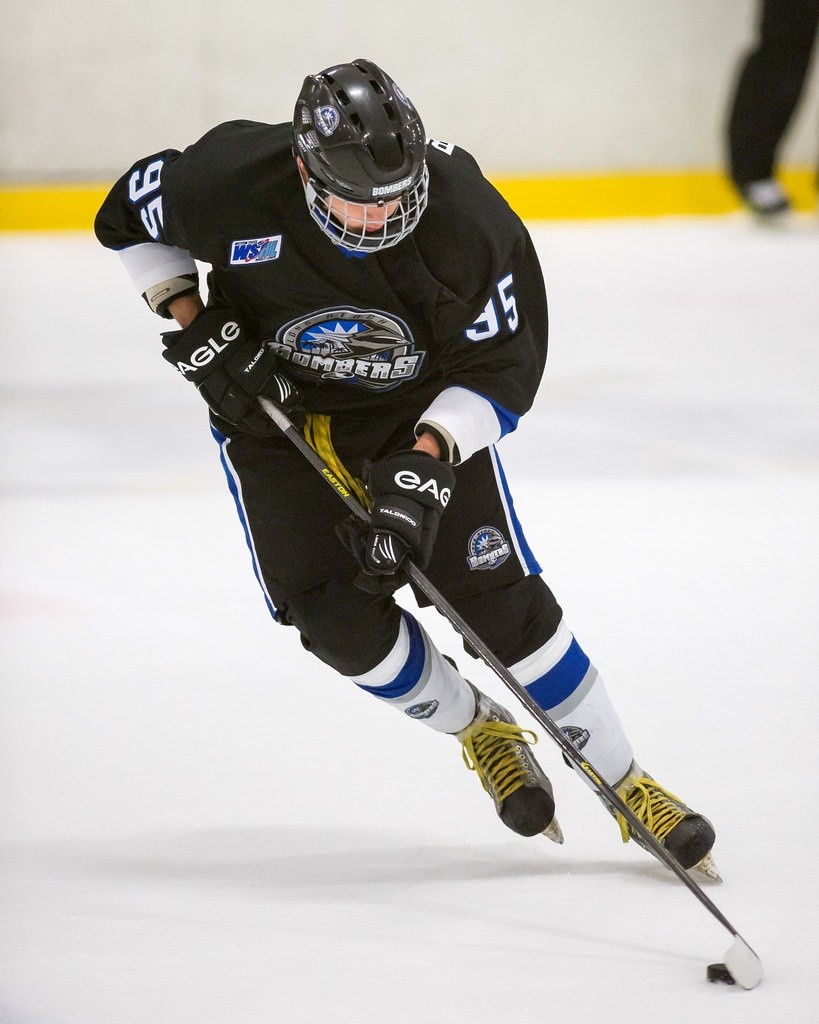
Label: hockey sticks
xmin=250 ymin=395 xmax=763 ymax=992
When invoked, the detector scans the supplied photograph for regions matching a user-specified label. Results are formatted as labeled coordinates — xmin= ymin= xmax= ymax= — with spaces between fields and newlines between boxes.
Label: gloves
xmin=337 ymin=450 xmax=456 ymax=598
xmin=161 ymin=305 xmax=307 ymax=438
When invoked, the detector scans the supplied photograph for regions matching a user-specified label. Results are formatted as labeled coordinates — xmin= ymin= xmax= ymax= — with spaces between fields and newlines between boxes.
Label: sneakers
xmin=594 ymin=757 xmax=721 ymax=881
xmin=445 ymin=678 xmax=565 ymax=846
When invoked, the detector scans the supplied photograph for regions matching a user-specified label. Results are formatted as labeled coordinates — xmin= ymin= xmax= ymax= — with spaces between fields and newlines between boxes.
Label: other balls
xmin=707 ymin=963 xmax=736 ymax=985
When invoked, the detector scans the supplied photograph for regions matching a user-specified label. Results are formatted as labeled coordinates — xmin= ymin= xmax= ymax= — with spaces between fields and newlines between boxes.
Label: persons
xmin=91 ymin=57 xmax=723 ymax=887
xmin=720 ymin=0 xmax=819 ymax=221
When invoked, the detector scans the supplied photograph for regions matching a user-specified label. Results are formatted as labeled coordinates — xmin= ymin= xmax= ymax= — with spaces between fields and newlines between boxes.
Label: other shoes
xmin=734 ymin=176 xmax=788 ymax=215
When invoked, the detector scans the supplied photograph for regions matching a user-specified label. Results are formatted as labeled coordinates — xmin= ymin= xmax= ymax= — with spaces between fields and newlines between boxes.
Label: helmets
xmin=291 ymin=58 xmax=427 ymax=207
